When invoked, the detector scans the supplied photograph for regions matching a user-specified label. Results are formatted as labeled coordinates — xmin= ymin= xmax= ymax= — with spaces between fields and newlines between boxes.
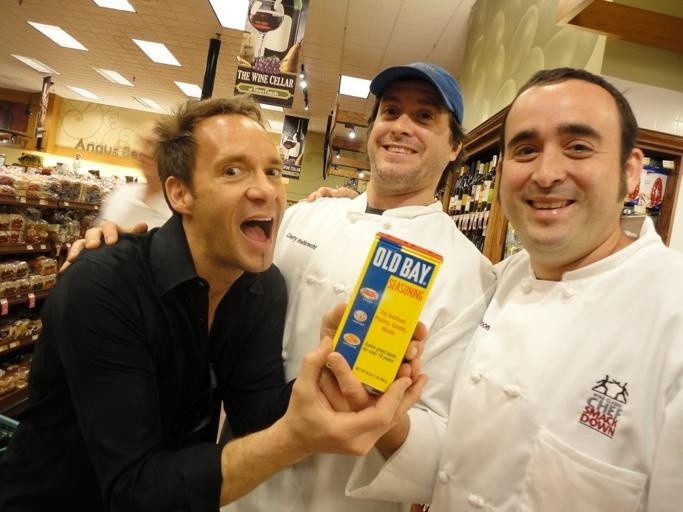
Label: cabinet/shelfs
xmin=441 ymin=103 xmax=512 ymax=257
xmin=490 ymin=126 xmax=683 ymax=265
xmin=0 ymin=193 xmax=100 ymax=416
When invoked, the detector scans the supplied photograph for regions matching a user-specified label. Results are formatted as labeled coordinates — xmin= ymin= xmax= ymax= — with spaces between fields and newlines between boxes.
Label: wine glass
xmin=247 ymin=1 xmax=283 ymax=69
xmin=281 ymin=133 xmax=295 ymax=166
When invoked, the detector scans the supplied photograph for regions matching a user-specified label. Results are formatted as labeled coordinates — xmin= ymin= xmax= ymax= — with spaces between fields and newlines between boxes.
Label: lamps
xmin=299 ymin=64 xmax=370 ymax=181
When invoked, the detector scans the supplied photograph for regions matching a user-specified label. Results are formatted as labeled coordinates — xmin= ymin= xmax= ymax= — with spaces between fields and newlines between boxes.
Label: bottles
xmin=290 ymin=118 xmax=304 ymax=160
xmin=446 ymin=155 xmax=498 ymax=252
xmin=260 ymin=0 xmax=302 ymax=63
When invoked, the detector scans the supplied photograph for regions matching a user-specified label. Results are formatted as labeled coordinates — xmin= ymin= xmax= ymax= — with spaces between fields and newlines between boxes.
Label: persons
xmin=58 ymin=61 xmax=499 ymax=510
xmin=312 ymin=68 xmax=683 ymax=509
xmin=1 ymin=95 xmax=431 ymax=511
xmin=95 ymin=126 xmax=173 ymax=231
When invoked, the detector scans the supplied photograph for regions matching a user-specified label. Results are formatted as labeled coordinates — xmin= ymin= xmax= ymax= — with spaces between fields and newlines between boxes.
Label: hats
xmin=370 ymin=63 xmax=462 ymax=124
